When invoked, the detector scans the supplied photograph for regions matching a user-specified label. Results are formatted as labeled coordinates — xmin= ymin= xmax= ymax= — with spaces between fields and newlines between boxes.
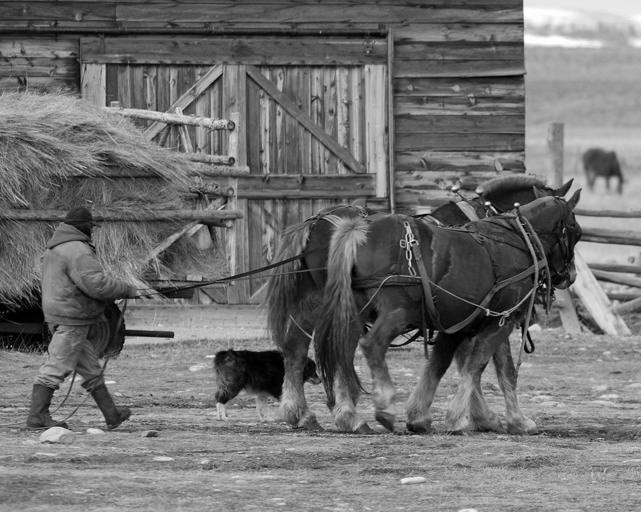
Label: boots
xmin=26 ymin=383 xmax=69 ymax=431
xmin=80 ymin=375 xmax=131 ymax=431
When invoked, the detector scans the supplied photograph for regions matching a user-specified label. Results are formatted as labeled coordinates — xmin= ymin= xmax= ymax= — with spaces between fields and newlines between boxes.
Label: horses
xmin=245 ymin=173 xmax=575 ymax=433
xmin=312 ymin=185 xmax=583 ymax=435
xmin=583 ymin=148 xmax=625 ymax=195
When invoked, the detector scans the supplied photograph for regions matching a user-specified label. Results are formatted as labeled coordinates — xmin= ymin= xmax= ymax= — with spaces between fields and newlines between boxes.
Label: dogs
xmin=213 ymin=347 xmax=322 ymax=423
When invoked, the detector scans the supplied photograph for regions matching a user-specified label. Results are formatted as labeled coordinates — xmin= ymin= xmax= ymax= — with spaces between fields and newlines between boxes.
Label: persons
xmin=25 ymin=207 xmax=139 ymax=432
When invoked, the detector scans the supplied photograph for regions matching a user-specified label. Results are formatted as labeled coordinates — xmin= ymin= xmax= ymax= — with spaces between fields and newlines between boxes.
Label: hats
xmin=64 ymin=207 xmax=101 ymax=227
